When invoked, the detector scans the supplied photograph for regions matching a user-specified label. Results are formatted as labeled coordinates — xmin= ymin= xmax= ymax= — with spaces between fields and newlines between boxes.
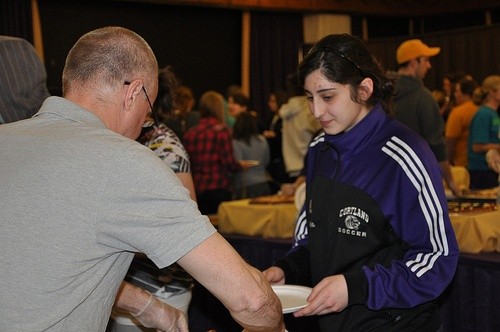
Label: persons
xmin=278 ymin=96 xmax=323 ymax=184
xmin=468 ymin=75 xmax=500 ymax=190
xmin=446 ymin=80 xmax=479 ymax=165
xmin=229 ymin=93 xmax=256 ymax=119
xmin=0 ymin=25 xmax=287 ymax=332
xmin=262 ymin=91 xmax=285 ymax=185
xmin=110 ymin=68 xmax=198 ymax=332
xmin=434 ymin=74 xmax=457 ymax=115
xmin=0 ymin=37 xmax=47 ymax=123
xmin=185 ymin=91 xmax=259 ymax=216
xmin=384 ymin=40 xmax=466 ymax=202
xmin=230 ymin=113 xmax=271 ymax=197
xmin=260 ymin=34 xmax=474 ymax=332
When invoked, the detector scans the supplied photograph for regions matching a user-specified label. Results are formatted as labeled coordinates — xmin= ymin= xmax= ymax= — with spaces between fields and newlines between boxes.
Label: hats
xmin=396 ymin=40 xmax=441 ymax=63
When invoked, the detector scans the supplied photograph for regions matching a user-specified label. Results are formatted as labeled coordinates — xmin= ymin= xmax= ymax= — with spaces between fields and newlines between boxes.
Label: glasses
xmin=124 ymin=81 xmax=159 ymax=129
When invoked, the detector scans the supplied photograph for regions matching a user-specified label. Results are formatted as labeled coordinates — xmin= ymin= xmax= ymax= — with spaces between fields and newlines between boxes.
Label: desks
xmin=218 ymin=187 xmax=500 ymax=254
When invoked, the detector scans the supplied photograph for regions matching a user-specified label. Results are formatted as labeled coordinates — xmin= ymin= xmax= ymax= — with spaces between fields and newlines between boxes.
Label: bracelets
xmin=130 ymin=291 xmax=153 ymax=318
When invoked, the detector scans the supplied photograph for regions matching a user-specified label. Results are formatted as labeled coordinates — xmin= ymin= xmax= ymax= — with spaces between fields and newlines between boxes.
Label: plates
xmin=270 ymin=285 xmax=314 ymax=314
xmin=239 ymin=160 xmax=259 ymax=165
